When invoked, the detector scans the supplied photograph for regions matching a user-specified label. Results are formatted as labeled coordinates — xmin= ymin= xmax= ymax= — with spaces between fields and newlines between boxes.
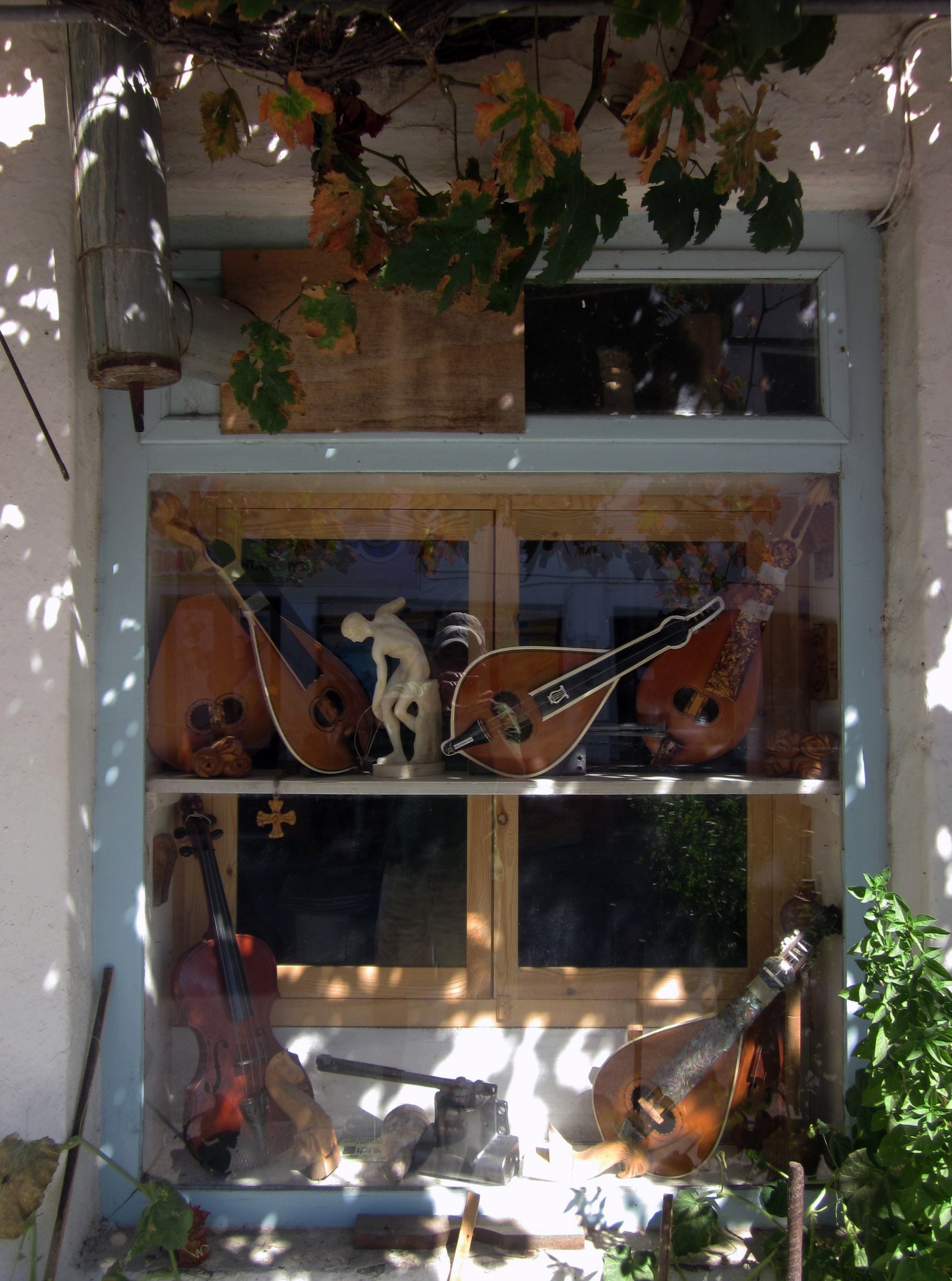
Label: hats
xmin=433 ymin=612 xmax=486 ymax=656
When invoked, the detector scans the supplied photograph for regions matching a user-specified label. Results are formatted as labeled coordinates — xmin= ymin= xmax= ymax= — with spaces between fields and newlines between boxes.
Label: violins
xmin=169 ymin=793 xmax=316 ymax=1177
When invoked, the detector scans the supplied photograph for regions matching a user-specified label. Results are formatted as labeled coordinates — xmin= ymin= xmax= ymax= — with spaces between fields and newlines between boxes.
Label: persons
xmin=371 ymin=611 xmax=526 ymax=967
xmin=341 ymin=597 xmax=430 ymax=767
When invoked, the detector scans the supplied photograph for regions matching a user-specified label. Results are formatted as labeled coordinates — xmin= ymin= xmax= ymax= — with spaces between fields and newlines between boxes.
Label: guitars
xmin=590 ymin=902 xmax=844 ymax=1179
xmin=439 ymin=595 xmax=726 ymax=780
xmin=635 ymin=476 xmax=838 ymax=775
xmin=149 ymin=490 xmax=374 ymax=775
